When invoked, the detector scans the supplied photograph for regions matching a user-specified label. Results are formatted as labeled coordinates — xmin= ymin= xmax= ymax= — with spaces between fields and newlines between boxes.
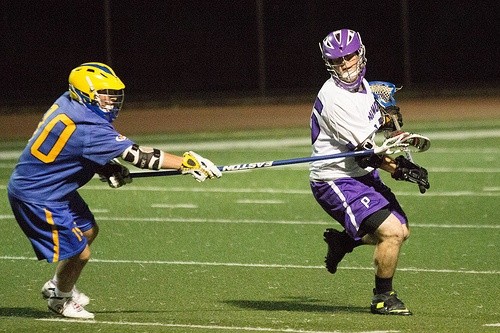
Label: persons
xmin=308 ymin=29 xmax=428 ymax=314
xmin=8 ymin=62 xmax=222 ymax=318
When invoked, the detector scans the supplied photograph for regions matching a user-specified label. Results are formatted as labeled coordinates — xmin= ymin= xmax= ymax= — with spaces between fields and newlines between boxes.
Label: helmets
xmin=69 ymin=62 xmax=125 ymax=123
xmin=319 ymin=29 xmax=367 ymax=94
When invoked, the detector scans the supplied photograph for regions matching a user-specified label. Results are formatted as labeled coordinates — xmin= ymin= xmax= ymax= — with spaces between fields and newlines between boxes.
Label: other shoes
xmin=48 ymin=291 xmax=94 ymax=319
xmin=323 ymin=228 xmax=353 ymax=274
xmin=370 ymin=288 xmax=413 ymax=315
xmin=41 ymin=280 xmax=90 ymax=308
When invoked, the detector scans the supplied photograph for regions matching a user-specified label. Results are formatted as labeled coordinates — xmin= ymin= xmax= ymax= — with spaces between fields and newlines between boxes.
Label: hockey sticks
xmin=369 ymin=80 xmax=415 ymax=163
xmin=96 ymin=133 xmax=431 ymax=182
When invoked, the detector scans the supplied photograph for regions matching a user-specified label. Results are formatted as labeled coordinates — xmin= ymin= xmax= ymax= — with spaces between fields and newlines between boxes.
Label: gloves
xmin=97 ymin=158 xmax=132 ymax=189
xmin=391 ymin=155 xmax=430 ymax=190
xmin=375 ymin=105 xmax=404 ymax=134
xmin=180 ymin=151 xmax=222 ymax=182
